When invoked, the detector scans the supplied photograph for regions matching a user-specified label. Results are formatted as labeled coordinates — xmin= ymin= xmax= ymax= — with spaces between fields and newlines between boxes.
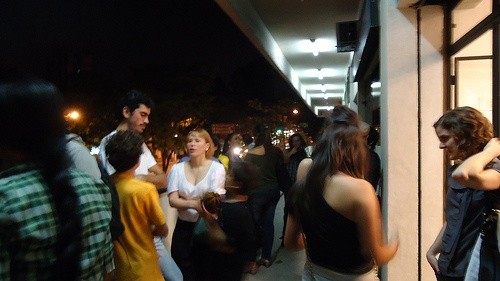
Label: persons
xmin=1 ymin=77 xmax=117 ymax=281
xmin=97 ymin=89 xmax=183 ymax=281
xmin=359 ymin=120 xmax=381 ymax=192
xmin=103 ymin=128 xmax=170 ymax=281
xmin=297 ymin=105 xmax=364 ymax=185
xmin=426 ymin=107 xmax=500 ymax=281
xmin=209 ymin=121 xmax=306 ymax=281
xmin=165 ymin=128 xmax=227 ymax=281
xmin=284 ymin=124 xmax=401 ymax=281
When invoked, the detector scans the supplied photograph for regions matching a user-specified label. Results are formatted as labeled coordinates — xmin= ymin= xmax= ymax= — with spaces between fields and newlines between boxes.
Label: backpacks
xmin=93 ymin=153 xmax=125 ymax=240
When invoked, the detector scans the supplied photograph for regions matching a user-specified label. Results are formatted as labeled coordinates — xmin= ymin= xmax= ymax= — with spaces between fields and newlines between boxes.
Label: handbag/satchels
xmin=463 ymin=190 xmax=500 ymax=281
